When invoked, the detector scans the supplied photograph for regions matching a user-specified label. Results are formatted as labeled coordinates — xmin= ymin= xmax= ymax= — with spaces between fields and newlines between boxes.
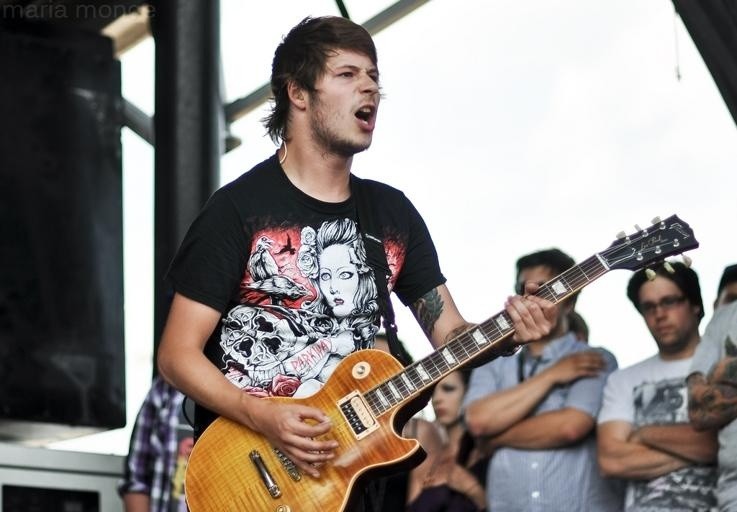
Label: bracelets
xmin=461 ymin=481 xmax=480 ymax=495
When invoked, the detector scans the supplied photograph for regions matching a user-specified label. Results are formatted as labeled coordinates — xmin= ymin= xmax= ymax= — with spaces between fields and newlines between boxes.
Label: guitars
xmin=182 ymin=210 xmax=700 ymax=512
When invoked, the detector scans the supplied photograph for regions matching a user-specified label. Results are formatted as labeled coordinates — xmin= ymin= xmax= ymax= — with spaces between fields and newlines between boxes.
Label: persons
xmin=294 ymin=217 xmax=384 ymax=358
xmin=459 ymin=250 xmax=622 ymax=512
xmin=592 ymin=261 xmax=719 ymax=510
xmin=708 ymin=261 xmax=737 ymax=309
xmin=396 ymin=364 xmax=487 ymax=512
xmin=685 ymin=291 xmax=737 ymax=512
xmin=116 ymin=370 xmax=201 ymax=512
xmin=154 ymin=13 xmax=556 ymax=510
